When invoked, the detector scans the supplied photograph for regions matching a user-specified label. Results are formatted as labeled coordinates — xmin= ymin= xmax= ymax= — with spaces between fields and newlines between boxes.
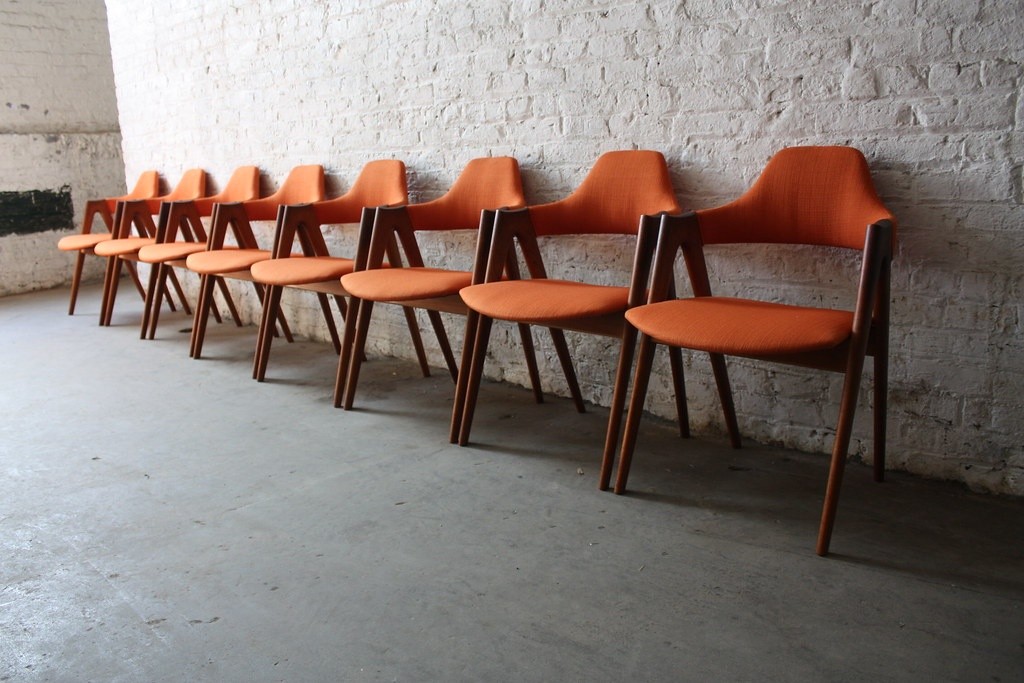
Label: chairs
xmin=103 ymin=168 xmax=222 ymax=340
xmin=256 ymin=159 xmax=430 ymax=409
xmin=457 ymin=150 xmax=690 ymax=490
xmin=343 ymin=156 xmax=544 ymax=445
xmin=146 ymin=165 xmax=280 ymax=357
xmin=57 ymin=168 xmax=177 ymax=326
xmin=193 ymin=164 xmax=341 ymax=379
xmin=613 ymin=146 xmax=895 ymax=557
